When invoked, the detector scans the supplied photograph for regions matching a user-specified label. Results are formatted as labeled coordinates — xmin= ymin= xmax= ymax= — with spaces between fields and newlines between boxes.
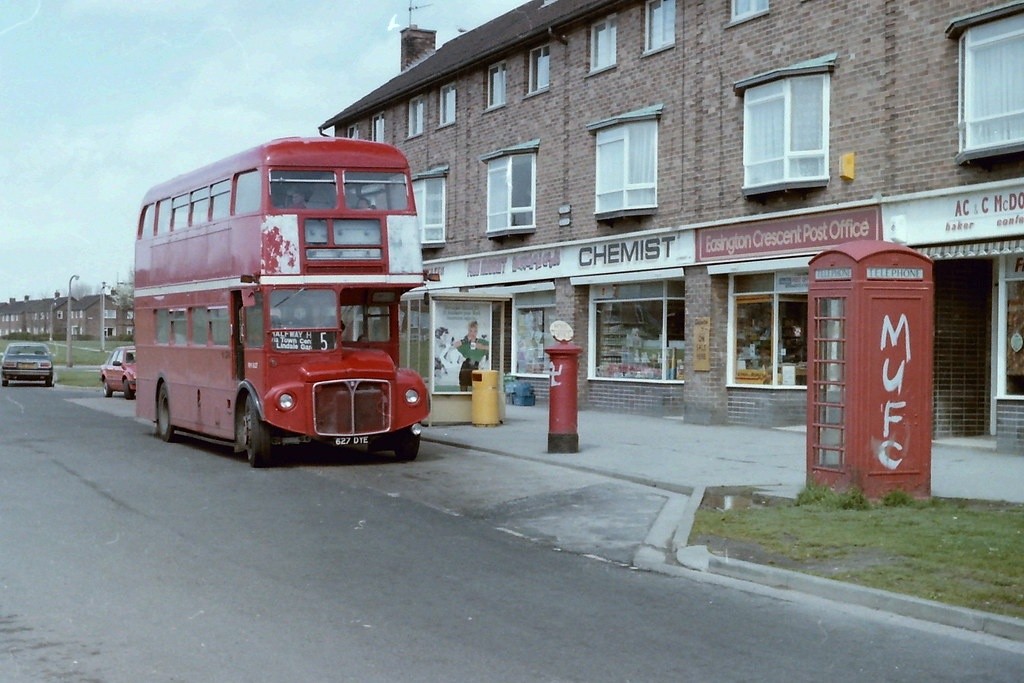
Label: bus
xmin=130 ymin=138 xmax=440 ymax=467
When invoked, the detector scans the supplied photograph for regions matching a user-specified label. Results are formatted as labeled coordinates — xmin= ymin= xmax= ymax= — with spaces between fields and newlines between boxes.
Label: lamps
xmin=558 ymin=205 xmax=572 ymax=241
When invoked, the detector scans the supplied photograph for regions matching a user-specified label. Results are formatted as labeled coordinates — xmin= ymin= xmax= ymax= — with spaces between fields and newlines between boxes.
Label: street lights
xmin=102 ymin=282 xmax=105 ymax=351
xmin=49 ymin=299 xmax=58 ymax=340
xmin=67 ymin=275 xmax=80 ymax=366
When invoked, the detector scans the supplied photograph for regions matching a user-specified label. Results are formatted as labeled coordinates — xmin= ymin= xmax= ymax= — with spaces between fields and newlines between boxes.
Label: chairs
xmin=126 ymin=353 xmax=135 ymax=363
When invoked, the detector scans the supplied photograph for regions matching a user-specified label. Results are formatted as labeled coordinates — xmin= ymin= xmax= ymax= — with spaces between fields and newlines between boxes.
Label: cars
xmin=100 ymin=345 xmax=137 ymax=400
xmin=0 ymin=343 xmax=55 ymax=387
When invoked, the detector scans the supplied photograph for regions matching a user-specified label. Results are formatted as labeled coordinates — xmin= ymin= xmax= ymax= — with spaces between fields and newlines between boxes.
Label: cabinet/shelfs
xmin=599 ymin=304 xmax=646 ymax=366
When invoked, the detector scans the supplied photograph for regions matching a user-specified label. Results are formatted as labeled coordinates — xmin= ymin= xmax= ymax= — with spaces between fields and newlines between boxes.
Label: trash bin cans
xmin=471 ymin=370 xmax=500 ymax=429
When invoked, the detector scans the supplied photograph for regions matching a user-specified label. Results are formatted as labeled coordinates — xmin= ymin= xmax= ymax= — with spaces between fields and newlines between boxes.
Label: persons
xmin=452 ymin=321 xmax=490 ymax=392
xmin=356 ymin=198 xmax=374 ymax=210
xmin=288 ymin=191 xmax=307 ymax=209
